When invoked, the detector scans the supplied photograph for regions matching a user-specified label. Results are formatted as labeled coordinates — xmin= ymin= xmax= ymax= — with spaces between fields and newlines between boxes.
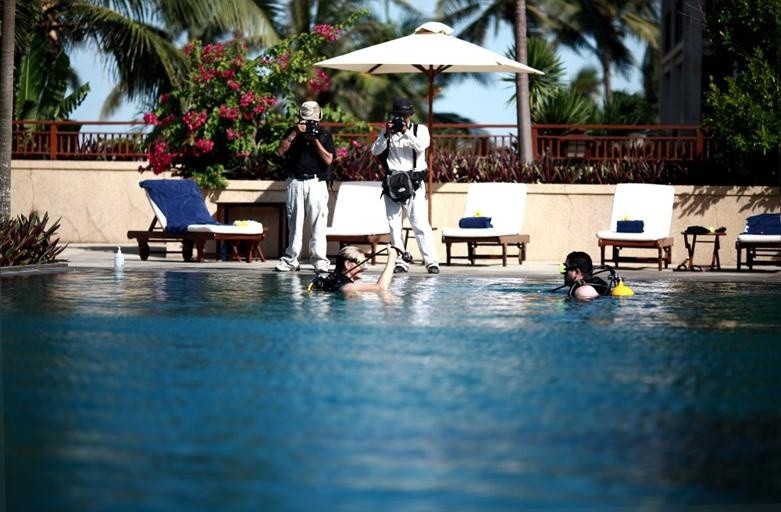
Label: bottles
xmin=113 ymin=247 xmax=125 ymax=272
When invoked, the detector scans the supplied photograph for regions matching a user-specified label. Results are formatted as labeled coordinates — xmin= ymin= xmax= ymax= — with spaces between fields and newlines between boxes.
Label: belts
xmin=288 ymin=174 xmax=321 ymax=181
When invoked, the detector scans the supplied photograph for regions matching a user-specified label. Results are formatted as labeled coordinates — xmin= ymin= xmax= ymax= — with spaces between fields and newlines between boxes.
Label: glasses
xmin=560 ymin=261 xmax=592 ymax=274
xmin=348 ymin=257 xmax=366 ymax=268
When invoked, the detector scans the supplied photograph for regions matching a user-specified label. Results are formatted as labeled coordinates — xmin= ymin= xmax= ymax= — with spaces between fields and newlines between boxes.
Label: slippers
xmin=673 ymin=265 xmax=689 ymax=271
xmin=690 ymin=265 xmax=702 ymax=272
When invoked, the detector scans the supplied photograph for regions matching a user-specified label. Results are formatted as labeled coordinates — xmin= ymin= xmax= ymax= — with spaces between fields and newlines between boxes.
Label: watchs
xmin=287 ymin=134 xmax=294 ymax=142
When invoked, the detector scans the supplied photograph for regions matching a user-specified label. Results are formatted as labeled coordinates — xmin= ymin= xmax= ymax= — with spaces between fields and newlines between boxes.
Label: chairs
xmin=596 ymin=183 xmax=676 ymax=270
xmin=126 ymin=180 xmax=266 ymax=263
xmin=734 ymin=213 xmax=781 ymax=272
xmin=441 ymin=182 xmax=532 ymax=266
xmin=326 ymin=180 xmax=403 ymax=265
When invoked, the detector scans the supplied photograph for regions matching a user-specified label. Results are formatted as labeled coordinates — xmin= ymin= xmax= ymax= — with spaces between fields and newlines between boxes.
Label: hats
xmin=299 ymin=100 xmax=323 ymax=121
xmin=391 ymin=98 xmax=416 ymax=114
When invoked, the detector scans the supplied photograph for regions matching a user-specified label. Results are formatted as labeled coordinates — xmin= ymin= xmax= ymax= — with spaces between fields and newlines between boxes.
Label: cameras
xmin=301 ymin=120 xmax=320 ymax=138
xmin=391 ymin=117 xmax=406 ymax=133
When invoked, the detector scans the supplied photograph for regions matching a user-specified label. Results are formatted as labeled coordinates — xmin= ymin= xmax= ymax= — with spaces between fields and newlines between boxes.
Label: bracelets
xmin=384 ymin=133 xmax=388 ymax=139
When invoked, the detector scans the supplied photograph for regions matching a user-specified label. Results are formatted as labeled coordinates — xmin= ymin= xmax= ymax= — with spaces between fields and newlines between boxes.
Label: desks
xmin=213 ymin=198 xmax=289 ymax=258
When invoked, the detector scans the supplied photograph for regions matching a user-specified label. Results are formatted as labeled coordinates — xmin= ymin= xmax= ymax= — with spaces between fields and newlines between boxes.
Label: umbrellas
xmin=311 ymin=19 xmax=546 ymax=229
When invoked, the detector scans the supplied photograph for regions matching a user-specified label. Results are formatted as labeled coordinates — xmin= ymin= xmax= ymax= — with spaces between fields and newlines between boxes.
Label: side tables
xmin=681 ymin=230 xmax=726 ymax=271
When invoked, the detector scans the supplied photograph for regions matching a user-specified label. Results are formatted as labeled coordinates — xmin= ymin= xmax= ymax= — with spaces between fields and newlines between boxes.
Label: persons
xmin=559 ymin=251 xmax=611 ymax=301
xmin=307 ymin=244 xmax=398 ymax=296
xmin=274 ymin=100 xmax=336 ymax=273
xmin=370 ymin=98 xmax=441 ymax=274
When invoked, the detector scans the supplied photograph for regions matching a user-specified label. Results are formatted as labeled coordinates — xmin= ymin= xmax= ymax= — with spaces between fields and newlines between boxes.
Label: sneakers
xmin=275 ymin=258 xmax=300 ymax=271
xmin=393 ymin=266 xmax=405 ymax=273
xmin=428 ymin=266 xmax=440 ymax=274
xmin=314 ymin=262 xmax=328 ymax=273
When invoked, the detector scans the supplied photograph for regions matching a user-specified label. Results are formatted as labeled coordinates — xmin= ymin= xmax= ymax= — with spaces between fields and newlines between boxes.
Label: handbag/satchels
xmin=685 ymin=225 xmax=710 ymax=235
xmin=380 ymin=169 xmax=429 ymax=202
